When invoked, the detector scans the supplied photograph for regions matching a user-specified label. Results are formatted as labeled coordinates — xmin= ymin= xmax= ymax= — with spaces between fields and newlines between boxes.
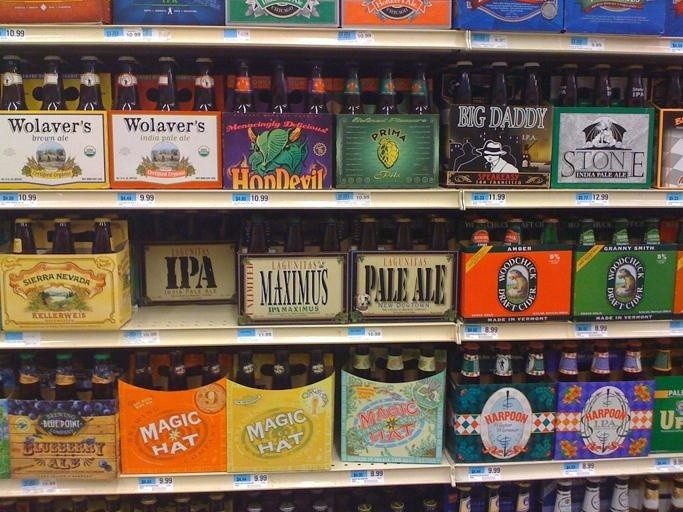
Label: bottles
xmin=0 ymin=55 xmax=683 ymax=115
xmin=17 ymin=335 xmax=683 ymax=402
xmin=12 ymin=211 xmax=683 ymax=254
xmin=0 ymin=475 xmax=682 ymax=512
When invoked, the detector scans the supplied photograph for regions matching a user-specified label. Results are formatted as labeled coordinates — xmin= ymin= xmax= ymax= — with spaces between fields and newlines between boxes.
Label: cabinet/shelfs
xmin=0 ymin=24 xmax=683 ymax=499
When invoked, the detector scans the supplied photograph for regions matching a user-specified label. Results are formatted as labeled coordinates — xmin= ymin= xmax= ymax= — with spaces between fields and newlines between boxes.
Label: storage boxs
xmin=0 ymin=352 xmax=683 ymax=479
xmin=0 ymin=74 xmax=683 ymax=190
xmin=1 ymin=213 xmax=683 ymax=332
xmin=0 ymin=0 xmax=683 ymax=35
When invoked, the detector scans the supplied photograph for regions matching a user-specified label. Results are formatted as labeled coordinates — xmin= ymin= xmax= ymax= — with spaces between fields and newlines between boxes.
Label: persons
xmin=452 ymin=139 xmax=520 ymax=173
